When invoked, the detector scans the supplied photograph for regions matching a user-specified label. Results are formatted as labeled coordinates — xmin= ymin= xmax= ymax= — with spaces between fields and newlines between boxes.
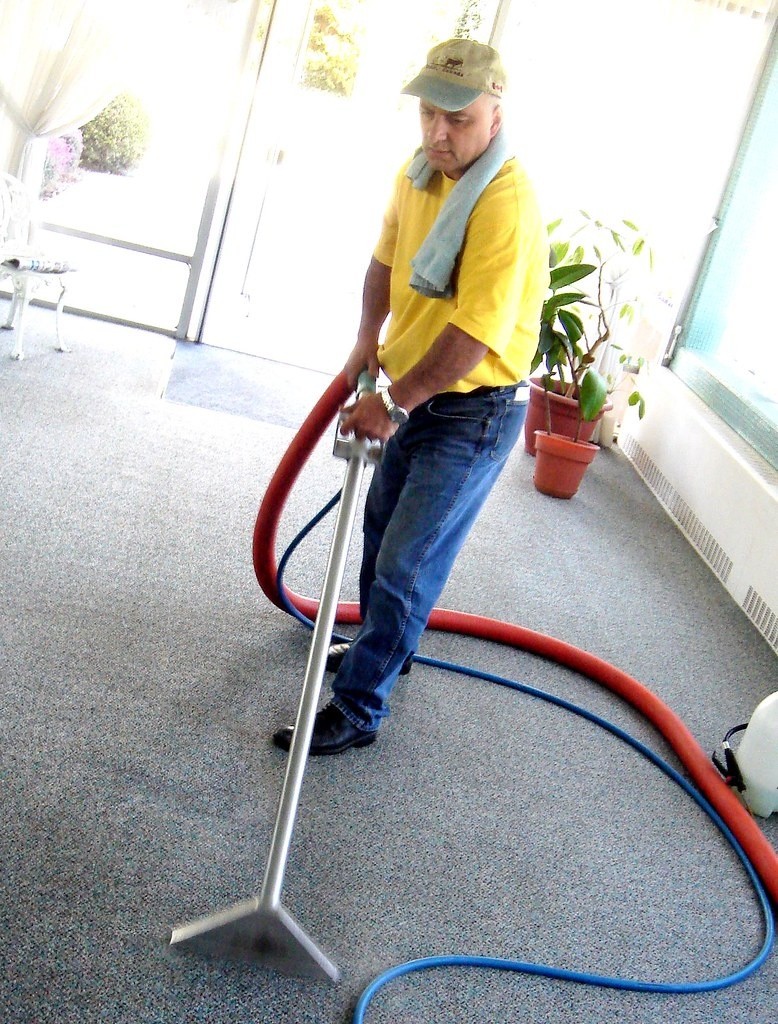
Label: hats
xmin=400 ymin=39 xmax=505 ymax=112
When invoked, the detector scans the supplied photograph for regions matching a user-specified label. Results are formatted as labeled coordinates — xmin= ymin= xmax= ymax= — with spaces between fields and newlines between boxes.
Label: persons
xmin=272 ymin=41 xmax=550 ymax=755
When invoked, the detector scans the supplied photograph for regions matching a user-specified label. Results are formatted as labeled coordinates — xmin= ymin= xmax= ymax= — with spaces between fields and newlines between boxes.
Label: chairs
xmin=0 ymin=172 xmax=80 ymax=360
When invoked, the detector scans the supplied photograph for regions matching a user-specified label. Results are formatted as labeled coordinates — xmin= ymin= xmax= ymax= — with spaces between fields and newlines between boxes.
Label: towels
xmin=403 ymin=118 xmax=518 ymax=301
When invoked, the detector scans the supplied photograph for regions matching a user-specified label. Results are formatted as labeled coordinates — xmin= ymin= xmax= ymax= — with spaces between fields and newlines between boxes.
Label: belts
xmin=435 ymin=386 xmax=506 ymax=398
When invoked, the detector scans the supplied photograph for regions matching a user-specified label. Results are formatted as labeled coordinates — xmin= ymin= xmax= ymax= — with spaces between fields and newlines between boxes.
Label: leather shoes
xmin=273 ymin=703 xmax=375 ymax=756
xmin=326 ymin=642 xmax=412 ymax=675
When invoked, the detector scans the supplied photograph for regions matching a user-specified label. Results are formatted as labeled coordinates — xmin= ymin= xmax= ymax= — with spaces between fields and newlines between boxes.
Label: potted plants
xmin=529 ymin=247 xmax=600 ymax=496
xmin=522 ymin=209 xmax=671 ymax=456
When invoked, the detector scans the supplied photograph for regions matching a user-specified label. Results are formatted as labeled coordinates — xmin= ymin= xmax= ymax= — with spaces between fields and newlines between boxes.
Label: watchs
xmin=381 ymin=386 xmax=409 ymax=424
